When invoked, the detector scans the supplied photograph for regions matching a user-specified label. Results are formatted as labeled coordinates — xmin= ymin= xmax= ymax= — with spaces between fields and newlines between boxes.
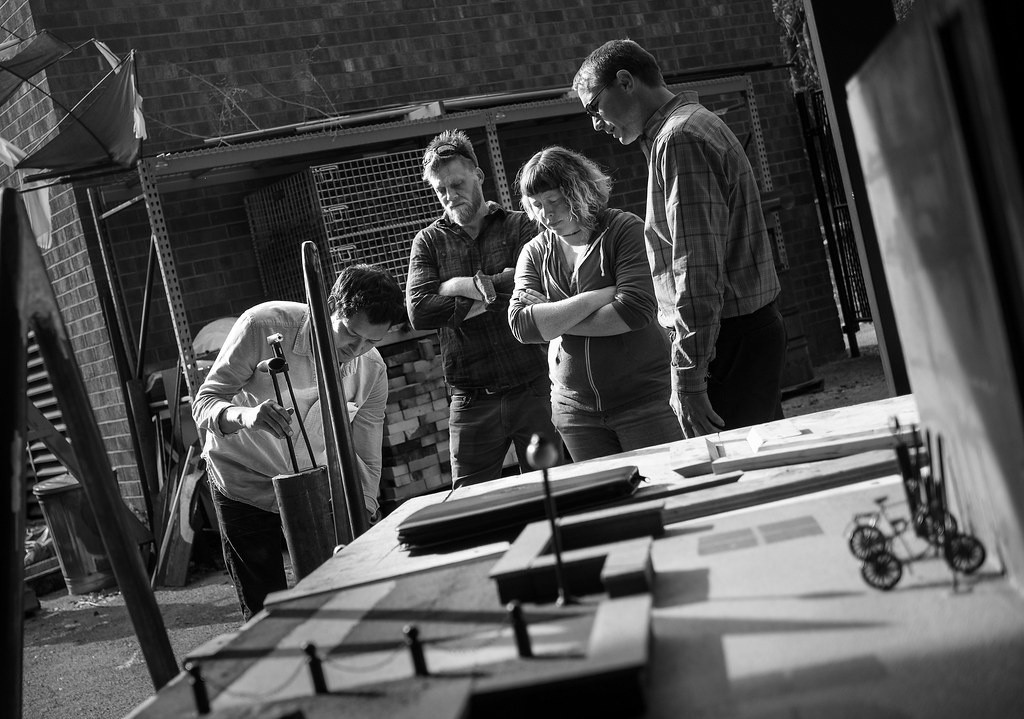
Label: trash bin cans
xmin=33 ymin=467 xmax=128 ymax=598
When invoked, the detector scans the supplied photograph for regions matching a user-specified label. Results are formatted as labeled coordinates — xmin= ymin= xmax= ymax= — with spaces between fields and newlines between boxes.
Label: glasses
xmin=586 ymin=77 xmax=616 ymax=117
xmin=421 ymin=145 xmax=472 ymax=169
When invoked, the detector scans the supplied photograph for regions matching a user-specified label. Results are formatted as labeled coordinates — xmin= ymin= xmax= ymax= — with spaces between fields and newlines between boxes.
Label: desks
xmin=124 ymin=393 xmax=1024 ymax=719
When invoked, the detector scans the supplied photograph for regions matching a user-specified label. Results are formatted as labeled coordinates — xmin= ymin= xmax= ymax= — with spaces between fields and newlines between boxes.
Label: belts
xmin=449 ymin=387 xmax=504 ymax=395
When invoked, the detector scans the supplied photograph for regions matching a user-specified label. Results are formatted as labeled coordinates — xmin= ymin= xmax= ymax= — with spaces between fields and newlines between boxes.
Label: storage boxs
xmin=243 ymin=147 xmax=444 ymax=306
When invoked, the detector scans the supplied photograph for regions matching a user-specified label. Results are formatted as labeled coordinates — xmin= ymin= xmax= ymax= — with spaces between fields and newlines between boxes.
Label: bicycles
xmin=842 ymin=478 xmax=986 ymax=588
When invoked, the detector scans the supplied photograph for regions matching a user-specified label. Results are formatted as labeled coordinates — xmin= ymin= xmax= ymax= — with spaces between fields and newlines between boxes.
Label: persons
xmin=571 ymin=39 xmax=787 ymax=438
xmin=405 ymin=128 xmax=567 ymax=486
xmin=191 ymin=263 xmax=405 ymax=619
xmin=508 ymin=147 xmax=688 ymax=464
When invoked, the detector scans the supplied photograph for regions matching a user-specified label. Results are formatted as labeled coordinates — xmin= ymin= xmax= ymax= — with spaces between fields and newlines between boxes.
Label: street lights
xmin=526 ymin=433 xmax=577 ymax=610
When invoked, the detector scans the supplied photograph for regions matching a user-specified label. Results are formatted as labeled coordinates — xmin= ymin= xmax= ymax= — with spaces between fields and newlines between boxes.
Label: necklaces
xmin=559 ymin=229 xmax=581 ymax=237
